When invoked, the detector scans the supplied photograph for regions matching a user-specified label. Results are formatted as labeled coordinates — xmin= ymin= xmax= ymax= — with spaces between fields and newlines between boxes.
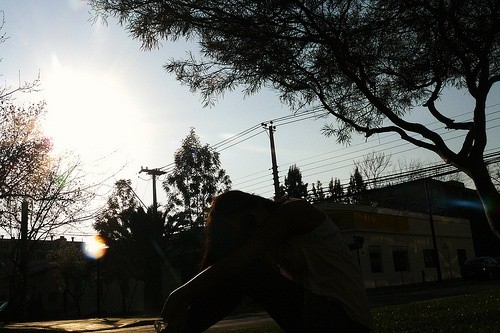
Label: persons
xmin=153 ymin=190 xmax=375 ymax=333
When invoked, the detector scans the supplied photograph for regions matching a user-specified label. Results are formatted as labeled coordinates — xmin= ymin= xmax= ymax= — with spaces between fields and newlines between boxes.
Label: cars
xmin=461 ymin=257 xmax=500 ymax=281
